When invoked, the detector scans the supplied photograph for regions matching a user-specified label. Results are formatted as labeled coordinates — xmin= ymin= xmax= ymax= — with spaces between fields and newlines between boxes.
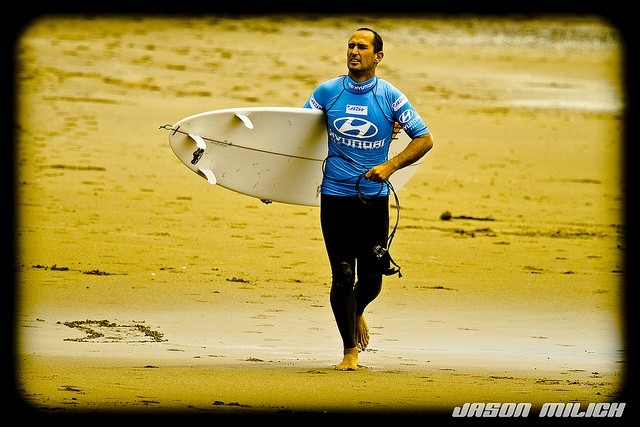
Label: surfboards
xmin=169 ymin=108 xmax=423 ymax=206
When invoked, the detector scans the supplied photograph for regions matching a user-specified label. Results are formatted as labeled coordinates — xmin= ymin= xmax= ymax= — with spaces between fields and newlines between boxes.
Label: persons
xmin=260 ymin=28 xmax=434 ymax=372
